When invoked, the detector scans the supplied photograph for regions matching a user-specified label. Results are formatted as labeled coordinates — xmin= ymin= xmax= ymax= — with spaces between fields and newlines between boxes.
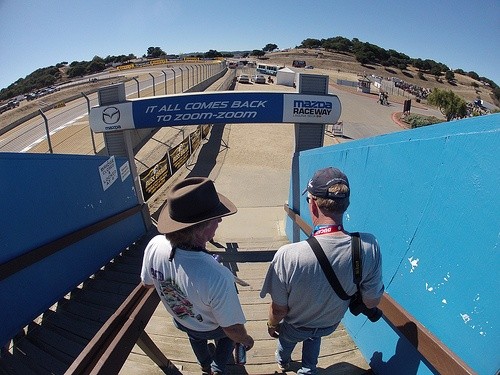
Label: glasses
xmin=305 ymin=197 xmax=318 ymax=204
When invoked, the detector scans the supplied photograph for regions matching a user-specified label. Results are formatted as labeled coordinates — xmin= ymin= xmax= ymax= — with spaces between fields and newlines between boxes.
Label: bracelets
xmin=266 ymin=322 xmax=276 ymax=328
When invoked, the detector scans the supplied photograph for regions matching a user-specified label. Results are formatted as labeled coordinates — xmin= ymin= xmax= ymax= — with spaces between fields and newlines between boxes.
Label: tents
xmin=277 ymin=67 xmax=295 ymax=87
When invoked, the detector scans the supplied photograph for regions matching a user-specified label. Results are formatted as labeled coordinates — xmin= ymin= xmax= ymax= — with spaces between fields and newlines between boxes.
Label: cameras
xmin=349 ymin=293 xmax=383 ymax=322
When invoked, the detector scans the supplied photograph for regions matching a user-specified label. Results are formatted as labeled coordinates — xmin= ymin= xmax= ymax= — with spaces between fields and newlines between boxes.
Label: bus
xmin=256 ymin=63 xmax=285 ymax=76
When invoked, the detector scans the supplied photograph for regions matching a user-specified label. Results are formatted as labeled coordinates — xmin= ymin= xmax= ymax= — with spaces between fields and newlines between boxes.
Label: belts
xmin=299 ymin=327 xmax=327 ymax=331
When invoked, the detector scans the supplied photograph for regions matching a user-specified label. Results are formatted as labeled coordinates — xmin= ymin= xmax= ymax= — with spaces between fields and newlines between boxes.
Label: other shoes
xmin=275 ymin=350 xmax=289 ymax=370
xmin=200 ymin=343 xmax=216 ymax=373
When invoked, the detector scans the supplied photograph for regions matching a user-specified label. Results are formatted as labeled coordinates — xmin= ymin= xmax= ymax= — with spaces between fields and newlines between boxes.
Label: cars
xmin=0 ymin=86 xmax=60 ymax=112
xmin=251 ymin=74 xmax=265 ymax=83
xmin=394 ymin=80 xmax=473 ymax=116
xmin=239 ymin=74 xmax=249 ymax=83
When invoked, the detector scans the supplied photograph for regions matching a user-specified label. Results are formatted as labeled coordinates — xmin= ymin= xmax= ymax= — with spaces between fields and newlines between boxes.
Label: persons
xmin=139 ymin=177 xmax=254 ymax=375
xmin=259 ymin=167 xmax=383 ymax=375
xmin=377 ymin=78 xmax=433 ymax=110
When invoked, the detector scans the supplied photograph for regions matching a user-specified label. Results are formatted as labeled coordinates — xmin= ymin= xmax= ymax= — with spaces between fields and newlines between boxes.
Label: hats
xmin=156 ymin=177 xmax=238 ymax=234
xmin=302 ymin=167 xmax=350 ymax=198
